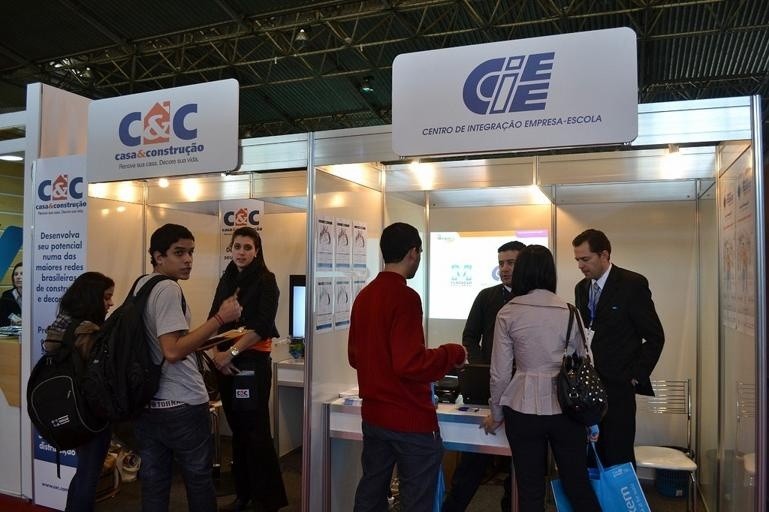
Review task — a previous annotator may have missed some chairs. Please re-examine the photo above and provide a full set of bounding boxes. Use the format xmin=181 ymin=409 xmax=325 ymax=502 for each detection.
xmin=634 ymin=377 xmax=712 ymax=512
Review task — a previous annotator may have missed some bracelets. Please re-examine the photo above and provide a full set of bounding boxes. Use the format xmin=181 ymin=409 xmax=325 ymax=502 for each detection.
xmin=212 ymin=312 xmax=226 ymax=329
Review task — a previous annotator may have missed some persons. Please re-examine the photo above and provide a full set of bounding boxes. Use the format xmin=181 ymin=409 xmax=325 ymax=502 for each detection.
xmin=439 ymin=240 xmax=528 ymax=512
xmin=347 ymin=221 xmax=468 ymax=511
xmin=477 ymin=244 xmax=601 ymax=512
xmin=572 ymin=229 xmax=665 ymax=474
xmin=206 ymin=226 xmax=290 ymax=511
xmin=35 ymin=270 xmax=120 ymax=511
xmin=0 ymin=262 xmax=24 ymax=328
xmin=127 ymin=222 xmax=243 ymax=512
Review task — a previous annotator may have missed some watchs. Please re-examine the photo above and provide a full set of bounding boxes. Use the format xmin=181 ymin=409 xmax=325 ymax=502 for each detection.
xmin=228 ymin=346 xmax=241 ymax=356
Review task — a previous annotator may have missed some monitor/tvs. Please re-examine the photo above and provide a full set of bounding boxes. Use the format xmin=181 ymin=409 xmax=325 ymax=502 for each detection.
xmin=459 ymin=364 xmax=492 ymax=406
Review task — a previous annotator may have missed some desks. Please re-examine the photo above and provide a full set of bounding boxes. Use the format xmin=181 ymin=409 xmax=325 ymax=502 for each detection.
xmin=274 ymin=357 xmax=305 ymax=463
xmin=324 ymin=396 xmax=518 ymax=512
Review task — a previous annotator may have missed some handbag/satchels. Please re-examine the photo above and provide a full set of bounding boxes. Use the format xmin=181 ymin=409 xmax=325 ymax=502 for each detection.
xmin=557 ymin=302 xmax=609 ymax=432
xmin=549 ymin=440 xmax=652 ymax=512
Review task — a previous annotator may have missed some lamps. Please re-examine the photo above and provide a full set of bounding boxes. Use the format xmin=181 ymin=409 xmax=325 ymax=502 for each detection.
xmin=295 ymin=27 xmax=308 ymax=45
xmin=80 ymin=59 xmax=95 ymax=80
xmin=362 ymin=75 xmax=374 ymax=94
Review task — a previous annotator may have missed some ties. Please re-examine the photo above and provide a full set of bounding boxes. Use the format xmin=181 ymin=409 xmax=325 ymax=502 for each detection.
xmin=589 ymin=282 xmax=600 ymax=329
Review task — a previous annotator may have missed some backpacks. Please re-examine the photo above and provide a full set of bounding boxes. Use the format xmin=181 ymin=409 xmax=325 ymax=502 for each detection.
xmin=80 ymin=274 xmax=187 ymax=426
xmin=25 ymin=319 xmax=112 ymax=450
